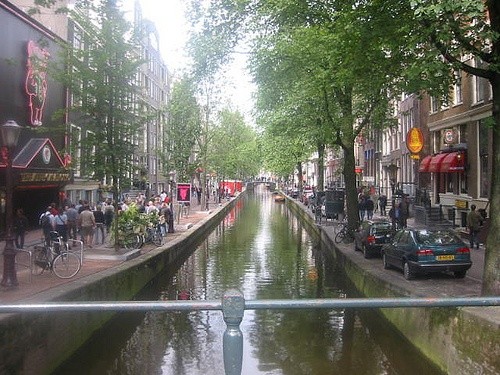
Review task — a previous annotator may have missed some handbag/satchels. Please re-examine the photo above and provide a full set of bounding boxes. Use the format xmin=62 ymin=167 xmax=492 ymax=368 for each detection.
xmin=473 ymin=224 xmax=480 ymax=231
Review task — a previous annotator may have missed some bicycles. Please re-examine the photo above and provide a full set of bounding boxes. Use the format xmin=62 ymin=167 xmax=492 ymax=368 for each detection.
xmin=124 ymin=219 xmax=165 ymax=250
xmin=334 ymin=222 xmax=359 ymax=244
xmin=30 ymin=231 xmax=82 ymax=279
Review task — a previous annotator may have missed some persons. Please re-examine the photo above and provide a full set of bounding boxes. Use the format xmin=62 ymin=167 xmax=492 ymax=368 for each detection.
xmin=359 ymin=192 xmax=373 ymax=221
xmin=118 ymin=192 xmax=171 ymax=236
xmin=13 ymin=208 xmax=29 ymax=248
xmin=388 ymin=197 xmax=409 ymax=229
xmin=468 ymin=204 xmax=483 ymax=249
xmin=378 ymin=195 xmax=387 ymax=216
xmin=39 ymin=199 xmax=115 ymax=256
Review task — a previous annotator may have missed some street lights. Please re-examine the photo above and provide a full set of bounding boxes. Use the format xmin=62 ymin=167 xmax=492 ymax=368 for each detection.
xmin=217 ymin=176 xmax=220 ymax=203
xmin=168 ymin=170 xmax=175 ymax=233
xmin=205 ymin=173 xmax=211 ymax=211
xmin=0 ymin=118 xmax=22 ymax=292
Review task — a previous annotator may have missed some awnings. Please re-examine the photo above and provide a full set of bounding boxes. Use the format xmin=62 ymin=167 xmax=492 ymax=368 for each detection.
xmin=418 ymin=151 xmax=465 ymax=172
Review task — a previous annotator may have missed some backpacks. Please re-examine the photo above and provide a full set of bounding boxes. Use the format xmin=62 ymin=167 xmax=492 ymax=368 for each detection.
xmin=41 ymin=213 xmax=51 ymax=228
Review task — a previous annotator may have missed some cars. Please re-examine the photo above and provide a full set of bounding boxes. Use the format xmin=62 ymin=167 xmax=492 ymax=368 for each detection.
xmin=281 ymin=183 xmax=345 ymax=219
xmin=380 ymin=224 xmax=473 ymax=280
xmin=353 ymin=219 xmax=400 ymax=259
